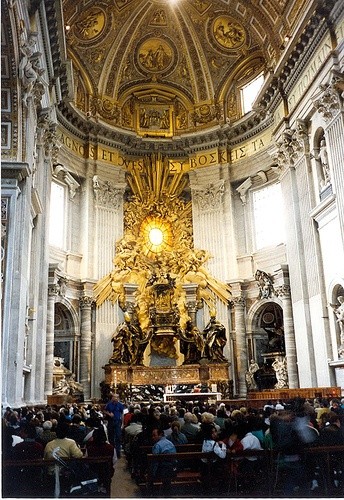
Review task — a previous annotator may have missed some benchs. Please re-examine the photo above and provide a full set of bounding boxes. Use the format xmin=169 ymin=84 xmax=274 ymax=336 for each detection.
xmin=2 ymin=435 xmax=344 ymax=499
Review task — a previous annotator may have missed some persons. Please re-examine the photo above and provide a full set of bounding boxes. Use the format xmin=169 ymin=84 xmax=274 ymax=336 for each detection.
xmin=13 ymin=28 xmax=344 ymax=209
xmin=105 ymin=309 xmax=231 ymax=367
xmin=1 ymin=403 xmax=112 ymax=498
xmin=122 ymin=396 xmax=344 ymax=498
xmin=103 ymin=393 xmax=126 ymax=460
xmin=51 ymin=373 xmax=84 ymax=396
xmin=246 ymin=268 xmax=344 ymax=391
